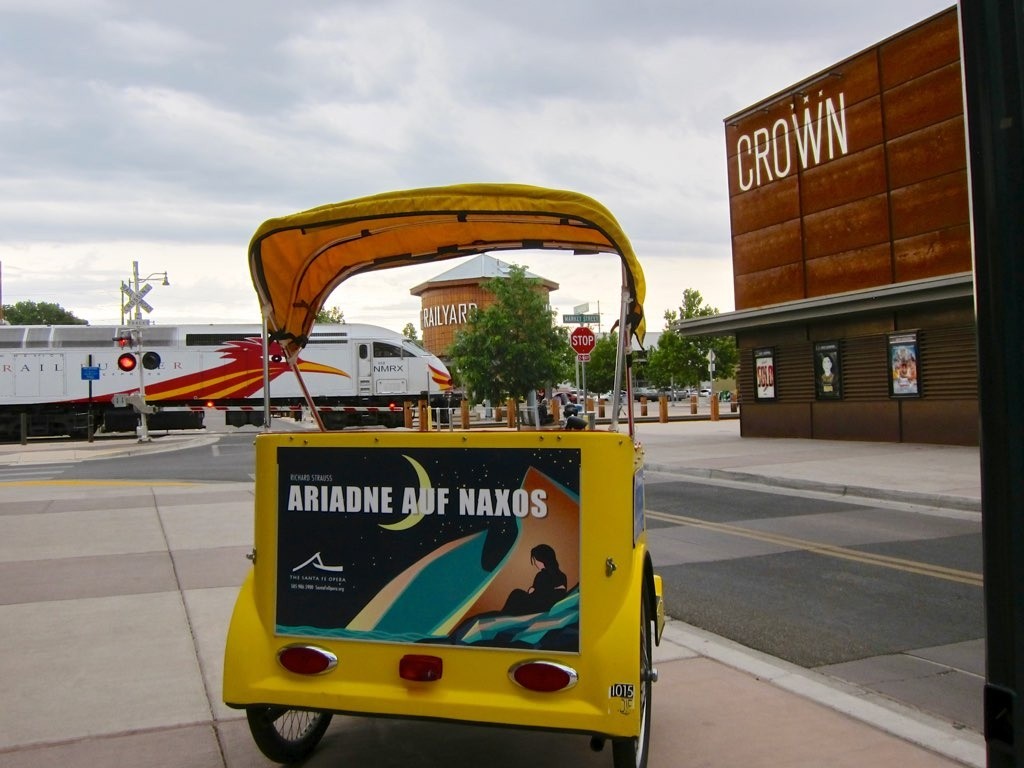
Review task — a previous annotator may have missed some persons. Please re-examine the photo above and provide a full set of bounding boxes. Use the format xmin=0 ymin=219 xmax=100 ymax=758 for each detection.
xmin=538 ymin=399 xmax=554 ymax=424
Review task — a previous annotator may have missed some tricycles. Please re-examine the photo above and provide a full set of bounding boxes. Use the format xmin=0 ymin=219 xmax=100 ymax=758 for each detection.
xmin=219 ymin=179 xmax=669 ymax=768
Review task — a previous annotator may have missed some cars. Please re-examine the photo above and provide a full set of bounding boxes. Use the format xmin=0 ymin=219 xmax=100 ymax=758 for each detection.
xmin=538 ymin=385 xmax=712 ymax=412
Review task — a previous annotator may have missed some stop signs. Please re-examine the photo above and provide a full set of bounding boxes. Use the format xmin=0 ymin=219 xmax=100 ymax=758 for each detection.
xmin=568 ymin=326 xmax=596 ymax=356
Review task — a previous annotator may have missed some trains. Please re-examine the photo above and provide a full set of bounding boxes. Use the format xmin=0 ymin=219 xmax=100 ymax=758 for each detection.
xmin=0 ymin=319 xmax=468 ymax=442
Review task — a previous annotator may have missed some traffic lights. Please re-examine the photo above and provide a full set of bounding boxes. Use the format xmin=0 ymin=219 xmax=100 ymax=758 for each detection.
xmin=141 ymin=350 xmax=161 ymax=371
xmin=116 ymin=353 xmax=137 ymax=372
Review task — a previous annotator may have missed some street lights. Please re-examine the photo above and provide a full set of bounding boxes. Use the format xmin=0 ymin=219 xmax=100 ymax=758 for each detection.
xmin=126 ymin=270 xmax=171 ymax=320
